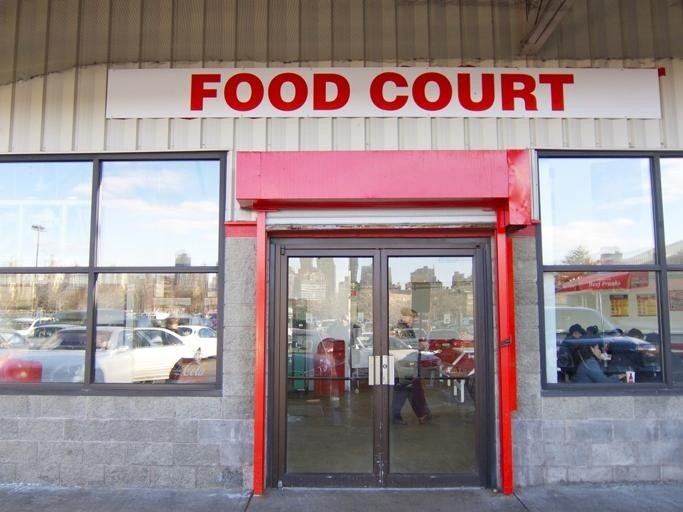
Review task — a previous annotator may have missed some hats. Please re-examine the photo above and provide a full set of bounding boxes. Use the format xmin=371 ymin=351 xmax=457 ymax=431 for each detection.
xmin=400 ymin=306 xmax=419 ymax=318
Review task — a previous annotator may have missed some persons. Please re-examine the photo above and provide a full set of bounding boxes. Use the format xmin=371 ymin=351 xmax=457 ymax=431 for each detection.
xmin=381 ymin=308 xmax=441 ymax=426
xmin=554 ymin=323 xmax=585 ymax=376
xmin=568 ymin=338 xmax=624 ymax=384
xmin=158 ymin=316 xmax=183 ymax=383
xmin=186 ymin=310 xmax=204 ymax=326
xmin=604 ymin=327 xmax=654 ymax=379
xmin=582 ymin=324 xmax=607 ymax=358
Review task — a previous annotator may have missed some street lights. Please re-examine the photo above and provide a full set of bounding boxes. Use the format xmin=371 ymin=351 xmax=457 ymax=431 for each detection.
xmin=32 ymin=226 xmax=43 ymax=284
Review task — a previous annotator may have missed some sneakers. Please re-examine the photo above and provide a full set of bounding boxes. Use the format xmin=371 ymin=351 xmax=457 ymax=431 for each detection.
xmin=418 ymin=413 xmax=439 ymax=424
xmin=392 ymin=415 xmax=408 ymax=426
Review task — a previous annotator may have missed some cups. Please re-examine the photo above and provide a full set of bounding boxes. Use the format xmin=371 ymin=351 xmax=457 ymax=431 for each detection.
xmin=626 ymin=371 xmax=635 ymax=384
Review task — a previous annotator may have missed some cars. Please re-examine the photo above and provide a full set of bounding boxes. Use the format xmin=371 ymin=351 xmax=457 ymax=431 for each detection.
xmin=1 ymin=308 xmax=217 ymax=383
xmin=556 ymin=307 xmax=661 ymax=380
xmin=287 ymin=309 xmax=476 ymax=393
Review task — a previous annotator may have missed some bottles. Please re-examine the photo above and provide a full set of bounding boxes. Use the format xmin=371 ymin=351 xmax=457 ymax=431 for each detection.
xmin=629 ymin=373 xmax=633 ymax=384
